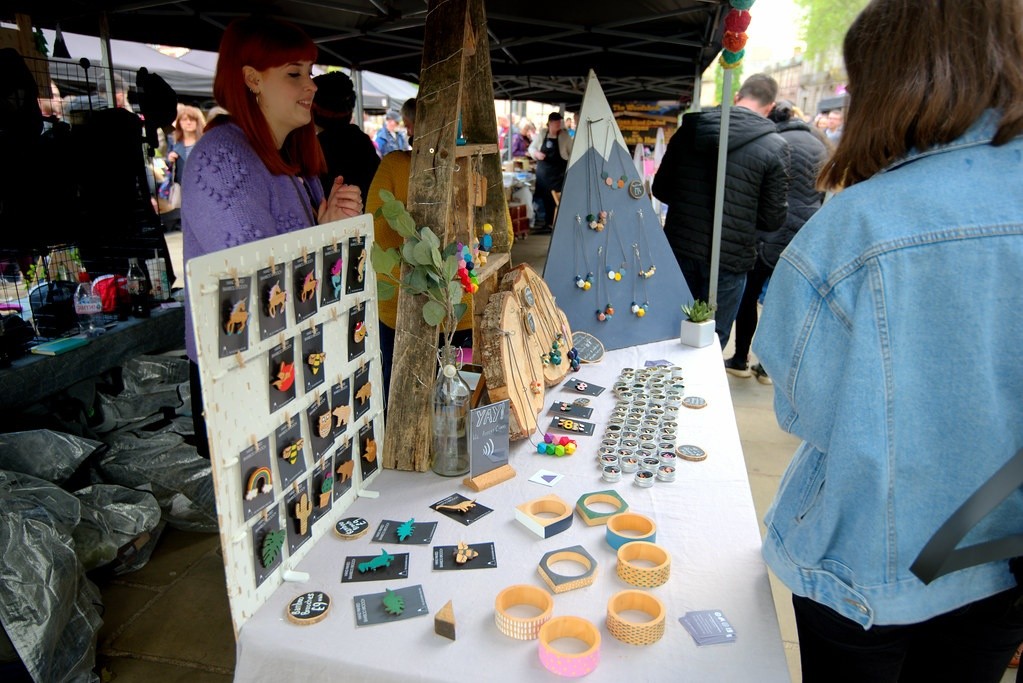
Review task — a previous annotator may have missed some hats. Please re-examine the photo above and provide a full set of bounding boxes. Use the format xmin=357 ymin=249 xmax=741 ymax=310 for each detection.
xmin=386 ymin=112 xmax=401 ymax=122
xmin=310 ymin=71 xmax=356 ymax=129
xmin=549 ymin=112 xmax=564 ymax=120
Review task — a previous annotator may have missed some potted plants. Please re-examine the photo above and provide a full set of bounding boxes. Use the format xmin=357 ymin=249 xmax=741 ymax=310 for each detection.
xmin=681 ymin=300 xmax=716 ymax=348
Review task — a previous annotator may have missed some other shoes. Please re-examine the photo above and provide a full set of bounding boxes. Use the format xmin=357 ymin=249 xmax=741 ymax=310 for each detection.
xmin=724 ymin=357 xmax=751 ymax=378
xmin=750 ymin=363 xmax=772 ymax=385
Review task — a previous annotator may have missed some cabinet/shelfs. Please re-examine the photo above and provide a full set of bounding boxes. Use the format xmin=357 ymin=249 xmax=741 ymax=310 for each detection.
xmin=381 ymin=0 xmax=514 ymax=474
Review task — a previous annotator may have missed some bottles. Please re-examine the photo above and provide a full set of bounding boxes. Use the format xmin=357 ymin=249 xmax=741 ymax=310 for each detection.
xmin=126 ymin=258 xmax=151 ymax=319
xmin=73 ymin=272 xmax=106 ymax=337
xmin=144 ymin=258 xmax=170 ymax=300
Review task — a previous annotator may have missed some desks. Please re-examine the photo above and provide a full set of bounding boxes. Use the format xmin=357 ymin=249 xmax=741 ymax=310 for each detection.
xmin=235 ymin=331 xmax=788 ymax=683
xmin=0 ymin=288 xmax=186 ymax=412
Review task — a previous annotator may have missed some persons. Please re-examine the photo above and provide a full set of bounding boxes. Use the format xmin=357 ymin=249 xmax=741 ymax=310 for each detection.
xmin=364 ymin=149 xmax=475 ymax=433
xmin=348 ymin=105 xmax=408 ymax=157
xmin=494 ymin=109 xmax=579 ymax=237
xmin=649 ymin=73 xmax=851 ymax=385
xmin=402 ymin=98 xmax=419 ymax=149
xmin=756 ymin=1 xmax=1023 ymax=683
xmin=310 ymin=72 xmax=381 ymax=206
xmin=180 ymin=19 xmax=361 ymax=467
xmin=37 ymin=65 xmax=234 ymax=235
